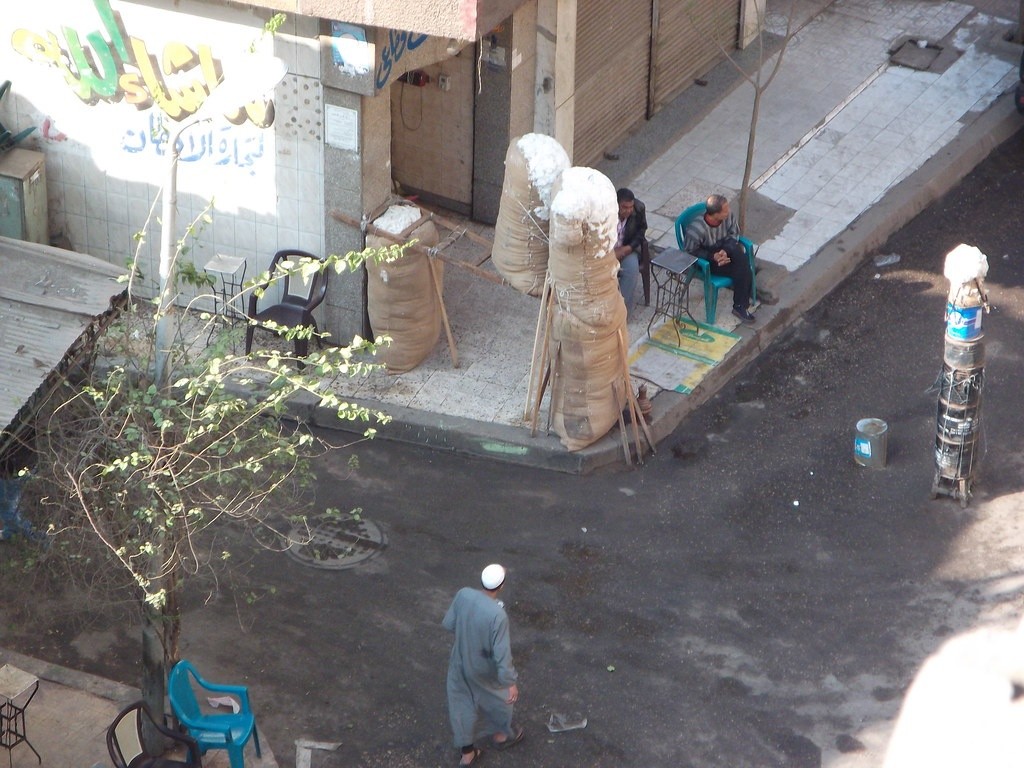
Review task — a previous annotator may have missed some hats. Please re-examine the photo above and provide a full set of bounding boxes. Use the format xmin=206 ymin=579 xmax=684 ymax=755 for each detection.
xmin=480 ymin=563 xmax=506 ymax=590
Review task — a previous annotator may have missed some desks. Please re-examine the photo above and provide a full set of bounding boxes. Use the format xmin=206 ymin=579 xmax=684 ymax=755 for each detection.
xmin=0 ymin=663 xmax=41 ymax=768
xmin=647 ymin=247 xmax=699 ymax=348
xmin=204 ymin=254 xmax=247 ymax=355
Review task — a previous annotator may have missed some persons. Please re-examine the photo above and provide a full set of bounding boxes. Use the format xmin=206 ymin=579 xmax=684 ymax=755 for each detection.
xmin=684 ymin=195 xmax=779 ymax=323
xmin=613 ymin=188 xmax=648 ymax=277
xmin=443 ymin=564 xmax=523 ymax=768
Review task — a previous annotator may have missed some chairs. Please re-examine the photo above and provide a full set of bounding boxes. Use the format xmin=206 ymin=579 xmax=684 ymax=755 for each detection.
xmin=168 ymin=660 xmax=261 ymax=768
xmin=675 ymin=202 xmax=756 ymax=324
xmin=106 ymin=701 xmax=203 ymax=768
xmin=246 ymin=250 xmax=329 ymax=376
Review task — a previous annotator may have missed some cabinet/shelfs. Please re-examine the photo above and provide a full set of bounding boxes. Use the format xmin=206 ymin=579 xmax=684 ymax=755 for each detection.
xmin=0 ymin=148 xmax=49 ymax=245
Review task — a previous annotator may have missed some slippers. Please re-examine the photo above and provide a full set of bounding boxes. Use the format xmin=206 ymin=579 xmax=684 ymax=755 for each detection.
xmin=459 ymin=748 xmax=483 ymax=767
xmin=493 ymin=723 xmax=526 ymax=750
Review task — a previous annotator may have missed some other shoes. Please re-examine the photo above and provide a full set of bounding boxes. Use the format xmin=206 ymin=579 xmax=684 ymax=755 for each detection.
xmin=732 ymin=303 xmax=756 ymax=322
